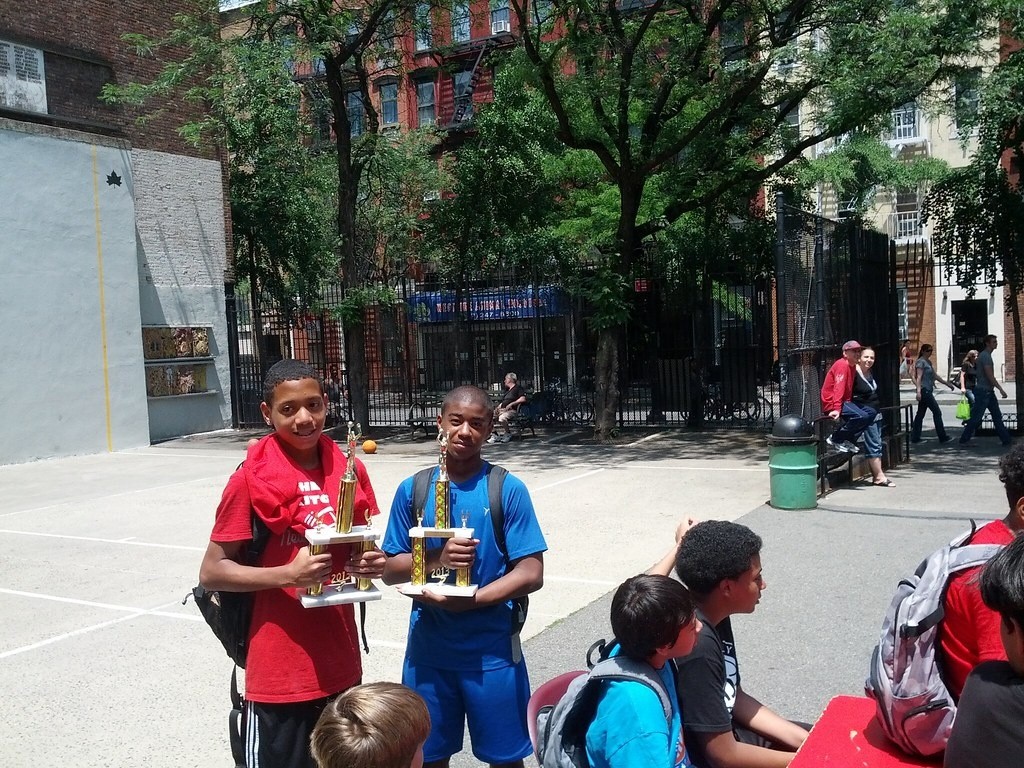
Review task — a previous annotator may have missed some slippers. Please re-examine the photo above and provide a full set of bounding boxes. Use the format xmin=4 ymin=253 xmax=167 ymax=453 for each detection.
xmin=872 ymin=478 xmax=896 ymax=487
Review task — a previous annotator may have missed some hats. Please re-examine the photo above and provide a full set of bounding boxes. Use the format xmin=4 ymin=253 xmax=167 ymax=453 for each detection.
xmin=841 ymin=340 xmax=864 ymax=351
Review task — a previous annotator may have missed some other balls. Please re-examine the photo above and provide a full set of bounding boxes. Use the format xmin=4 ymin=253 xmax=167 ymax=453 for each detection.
xmin=362 ymin=440 xmax=377 ymax=453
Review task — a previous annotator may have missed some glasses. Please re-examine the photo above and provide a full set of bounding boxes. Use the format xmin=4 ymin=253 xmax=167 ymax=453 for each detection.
xmin=926 ymin=349 xmax=933 ymax=352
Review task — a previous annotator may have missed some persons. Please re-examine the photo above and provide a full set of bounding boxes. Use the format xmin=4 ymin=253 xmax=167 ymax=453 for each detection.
xmin=322 ymin=364 xmax=348 ymax=421
xmin=200 ymin=358 xmax=385 ymax=768
xmin=437 ymin=429 xmax=449 ymax=478
xmin=381 ymin=387 xmax=548 ymax=768
xmin=911 ymin=344 xmax=954 ymax=443
xmin=958 ymin=335 xmax=1017 ymax=446
xmin=821 ymin=340 xmax=896 ymax=487
xmin=899 ymin=339 xmax=917 ymax=386
xmin=309 ymin=682 xmax=431 ymax=768
xmin=585 ymin=512 xmax=810 ymax=768
xmin=933 ymin=440 xmax=1024 ymax=708
xmin=486 ymin=373 xmax=526 ymax=444
xmin=959 ymin=350 xmax=979 ymax=427
xmin=346 ymin=421 xmax=362 ymax=473
xmin=944 ymin=530 xmax=1024 ymax=768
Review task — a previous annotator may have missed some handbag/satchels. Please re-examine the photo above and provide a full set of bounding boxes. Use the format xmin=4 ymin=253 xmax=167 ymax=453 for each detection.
xmin=899 ymin=357 xmax=908 ymax=376
xmin=951 ymin=361 xmax=966 ymax=390
xmin=956 ymin=391 xmax=971 ymax=420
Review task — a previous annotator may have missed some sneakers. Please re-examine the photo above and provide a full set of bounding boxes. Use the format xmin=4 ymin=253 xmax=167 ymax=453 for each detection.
xmin=501 ymin=431 xmax=512 ymax=443
xmin=826 ymin=434 xmax=860 ymax=454
xmin=487 ymin=433 xmax=500 ymax=443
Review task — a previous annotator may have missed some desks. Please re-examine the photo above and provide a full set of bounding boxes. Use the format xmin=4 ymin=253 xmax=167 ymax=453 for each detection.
xmin=789 ymin=693 xmax=943 ymax=768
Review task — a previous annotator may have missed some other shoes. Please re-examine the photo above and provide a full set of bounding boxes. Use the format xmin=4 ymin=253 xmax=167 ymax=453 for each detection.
xmin=911 ymin=440 xmax=927 ymax=444
xmin=939 ymin=436 xmax=955 ymax=443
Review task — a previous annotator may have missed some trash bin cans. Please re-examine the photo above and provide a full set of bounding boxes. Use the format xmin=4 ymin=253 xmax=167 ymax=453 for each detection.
xmin=765 ymin=413 xmax=819 ymax=511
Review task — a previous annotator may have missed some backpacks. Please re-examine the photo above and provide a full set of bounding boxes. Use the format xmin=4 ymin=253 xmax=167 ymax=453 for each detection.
xmin=864 ymin=519 xmax=1011 ymax=755
xmin=536 ymin=639 xmax=678 ymax=768
xmin=180 ymin=460 xmax=369 ymax=669
xmin=411 ymin=464 xmax=530 ymax=634
